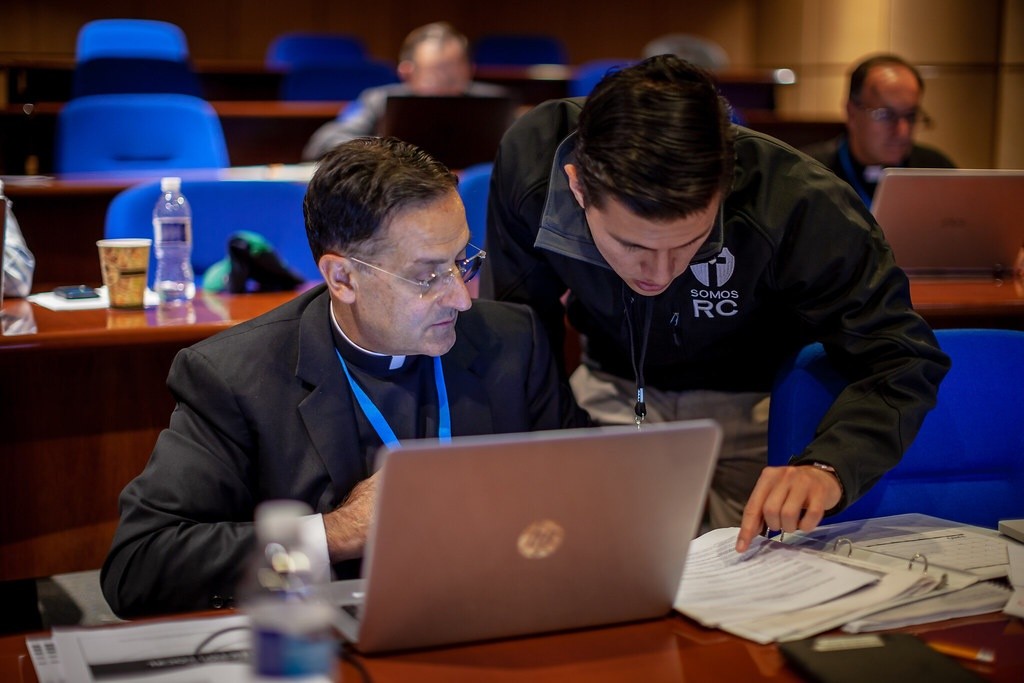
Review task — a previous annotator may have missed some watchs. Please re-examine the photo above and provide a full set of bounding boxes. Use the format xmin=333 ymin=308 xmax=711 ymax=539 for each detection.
xmin=811 ymin=461 xmax=844 ymax=498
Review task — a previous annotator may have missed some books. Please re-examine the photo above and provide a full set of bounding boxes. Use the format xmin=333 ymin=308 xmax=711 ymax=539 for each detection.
xmin=55 ymin=285 xmax=100 ymax=300
xmin=671 ymin=512 xmax=1024 ymax=683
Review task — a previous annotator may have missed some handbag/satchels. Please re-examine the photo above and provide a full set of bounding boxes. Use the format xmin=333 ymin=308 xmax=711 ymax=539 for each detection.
xmin=201 ymin=231 xmax=305 ymax=293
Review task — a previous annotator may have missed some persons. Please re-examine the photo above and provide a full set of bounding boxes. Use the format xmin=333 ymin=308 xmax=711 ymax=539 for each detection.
xmin=97 ymin=135 xmax=602 ymax=619
xmin=482 ymin=51 xmax=952 ymax=553
xmin=302 ymin=21 xmax=508 ymax=161
xmin=813 ymin=54 xmax=959 ymax=210
xmin=0 ymin=180 xmax=36 ymax=298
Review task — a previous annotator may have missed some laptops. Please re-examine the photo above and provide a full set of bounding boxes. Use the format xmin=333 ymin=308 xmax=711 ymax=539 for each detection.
xmin=307 ymin=418 xmax=723 ymax=655
xmin=864 ymin=168 xmax=1024 ymax=279
xmin=380 ymin=93 xmax=512 ymax=177
xmin=710 ymin=80 xmax=777 ymax=131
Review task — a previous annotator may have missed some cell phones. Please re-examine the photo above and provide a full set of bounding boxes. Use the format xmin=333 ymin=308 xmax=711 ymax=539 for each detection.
xmin=55 ymin=282 xmax=97 ymax=300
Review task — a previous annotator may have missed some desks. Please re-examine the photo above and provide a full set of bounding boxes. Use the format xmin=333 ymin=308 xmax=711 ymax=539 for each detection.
xmin=0 ymin=180 xmax=140 ymax=286
xmin=1 ymin=274 xmax=1024 ymax=545
xmin=0 ymin=101 xmax=543 ymax=181
xmin=1 ymin=601 xmax=1024 ymax=683
xmin=0 ymin=52 xmax=587 ymax=102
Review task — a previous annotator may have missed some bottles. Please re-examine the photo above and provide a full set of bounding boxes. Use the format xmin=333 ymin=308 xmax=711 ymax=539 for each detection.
xmin=152 ymin=177 xmax=195 ymax=310
xmin=248 ymin=498 xmax=337 ymax=683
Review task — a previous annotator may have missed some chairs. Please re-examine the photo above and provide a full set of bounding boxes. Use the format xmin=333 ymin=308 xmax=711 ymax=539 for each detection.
xmin=767 ymin=329 xmax=1024 ymax=538
xmin=58 ymin=21 xmax=737 ymax=292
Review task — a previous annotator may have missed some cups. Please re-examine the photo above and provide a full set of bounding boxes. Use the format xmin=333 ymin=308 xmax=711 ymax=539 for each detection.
xmin=96 ymin=238 xmax=152 ymax=310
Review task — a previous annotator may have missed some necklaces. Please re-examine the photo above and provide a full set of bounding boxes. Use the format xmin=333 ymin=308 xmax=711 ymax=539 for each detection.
xmin=621 ymin=275 xmax=655 ymax=424
xmin=840 ymin=136 xmax=872 ymax=210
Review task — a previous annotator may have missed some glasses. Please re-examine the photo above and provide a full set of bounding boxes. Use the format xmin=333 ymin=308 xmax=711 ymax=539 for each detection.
xmin=852 ymin=101 xmax=920 ymax=124
xmin=324 ymin=243 xmax=486 ymax=301
